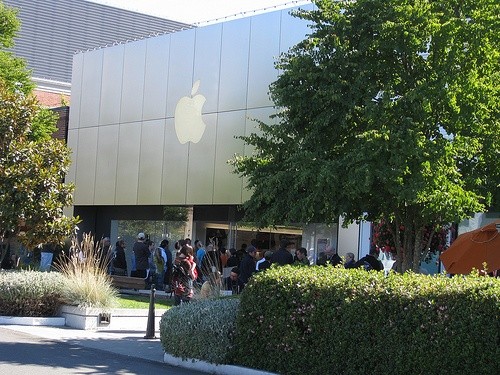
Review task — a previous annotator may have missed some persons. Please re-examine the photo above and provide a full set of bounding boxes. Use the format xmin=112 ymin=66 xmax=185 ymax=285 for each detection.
xmin=70 ymin=232 xmax=356 ymax=306
xmin=356 ymin=245 xmax=383 ymax=271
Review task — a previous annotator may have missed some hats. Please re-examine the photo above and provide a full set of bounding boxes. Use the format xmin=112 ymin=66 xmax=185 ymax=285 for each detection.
xmin=138 ymin=232 xmax=145 ymax=238
xmin=248 ymin=246 xmax=256 ymax=251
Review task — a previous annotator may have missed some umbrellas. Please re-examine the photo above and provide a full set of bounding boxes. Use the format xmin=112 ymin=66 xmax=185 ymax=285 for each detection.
xmin=440 ymin=224 xmax=500 ymax=277
